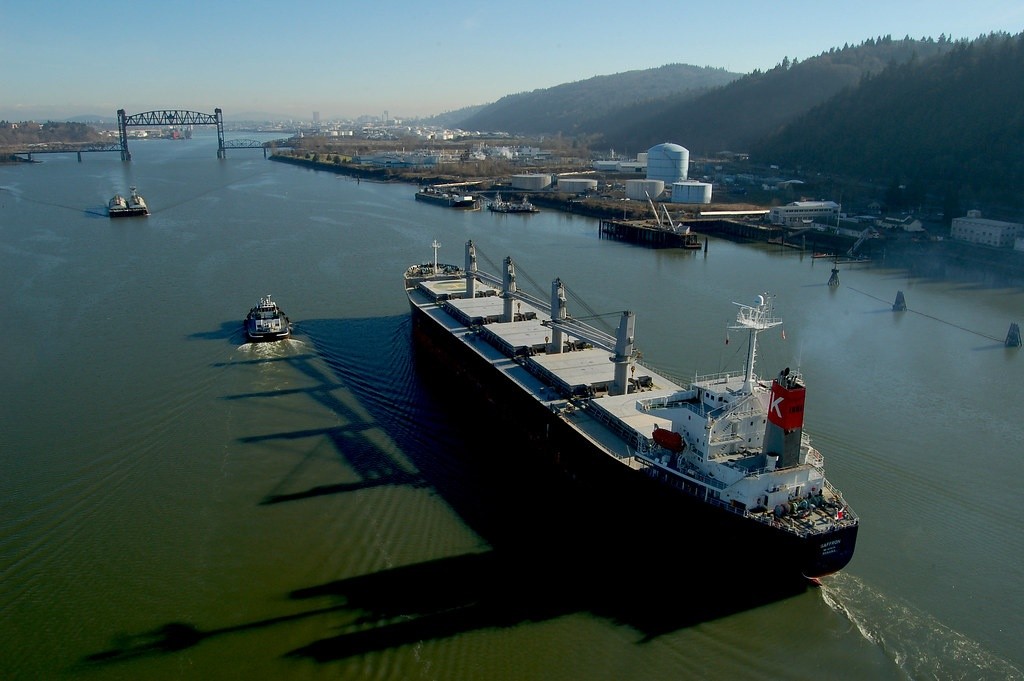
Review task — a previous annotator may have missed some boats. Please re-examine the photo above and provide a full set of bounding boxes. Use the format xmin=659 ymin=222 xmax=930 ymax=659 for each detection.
xmin=487 ymin=191 xmax=540 ymax=214
xmin=244 ymin=295 xmax=292 ymax=342
xmin=108 ymin=187 xmax=149 ymax=216
xmin=415 ymin=185 xmax=476 ymax=207
xmin=403 ymin=240 xmax=860 ymax=578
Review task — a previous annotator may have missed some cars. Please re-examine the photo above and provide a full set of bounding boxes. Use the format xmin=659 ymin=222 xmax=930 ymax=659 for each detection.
xmin=37 ymin=143 xmax=47 ymax=147
xmin=868 ymin=231 xmax=880 ymax=239
xmin=28 ymin=144 xmax=35 ymax=148
